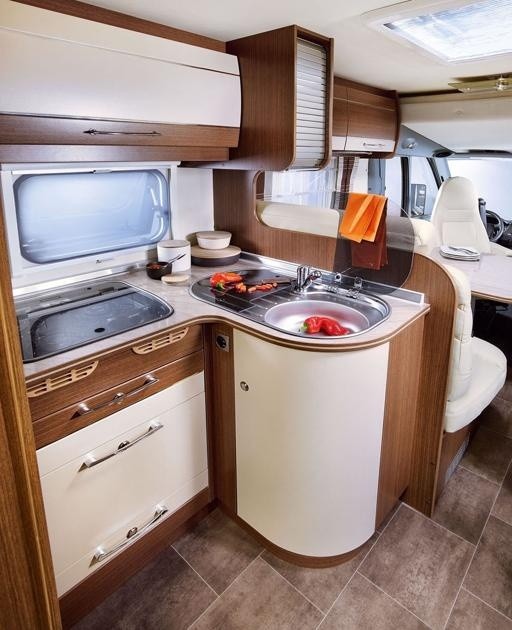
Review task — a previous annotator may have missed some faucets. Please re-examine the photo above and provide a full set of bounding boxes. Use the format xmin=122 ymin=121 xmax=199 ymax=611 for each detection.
xmin=298 ymin=266 xmax=321 ymax=290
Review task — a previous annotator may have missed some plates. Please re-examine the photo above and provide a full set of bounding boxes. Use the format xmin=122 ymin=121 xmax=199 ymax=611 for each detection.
xmin=439 ymin=244 xmax=480 ymax=263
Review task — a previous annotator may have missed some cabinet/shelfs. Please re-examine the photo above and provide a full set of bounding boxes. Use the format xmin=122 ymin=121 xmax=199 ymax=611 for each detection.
xmin=332 ymin=86 xmax=398 ymax=153
xmin=1 ymin=2 xmax=245 ymax=149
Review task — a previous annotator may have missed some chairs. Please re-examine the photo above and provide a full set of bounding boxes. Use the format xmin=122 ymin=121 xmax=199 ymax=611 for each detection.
xmin=384 ymin=179 xmax=512 ymax=436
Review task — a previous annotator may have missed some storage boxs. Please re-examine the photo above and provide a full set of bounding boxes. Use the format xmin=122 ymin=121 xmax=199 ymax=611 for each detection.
xmin=230 ymin=329 xmax=394 ymax=559
xmin=34 ymin=350 xmax=213 ymax=605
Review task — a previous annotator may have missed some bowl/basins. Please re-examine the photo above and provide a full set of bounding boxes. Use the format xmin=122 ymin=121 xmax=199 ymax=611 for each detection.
xmin=146 ymin=262 xmax=171 ymax=280
xmin=197 ymin=236 xmax=232 ymax=251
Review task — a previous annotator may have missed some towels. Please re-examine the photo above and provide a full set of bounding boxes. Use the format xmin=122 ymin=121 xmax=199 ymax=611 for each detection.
xmin=337 ymin=193 xmax=391 ymax=272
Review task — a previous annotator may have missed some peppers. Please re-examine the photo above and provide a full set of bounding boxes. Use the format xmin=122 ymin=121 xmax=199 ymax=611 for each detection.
xmin=209 ymin=271 xmax=242 ymax=290
xmin=299 ymin=316 xmax=348 ymax=335
